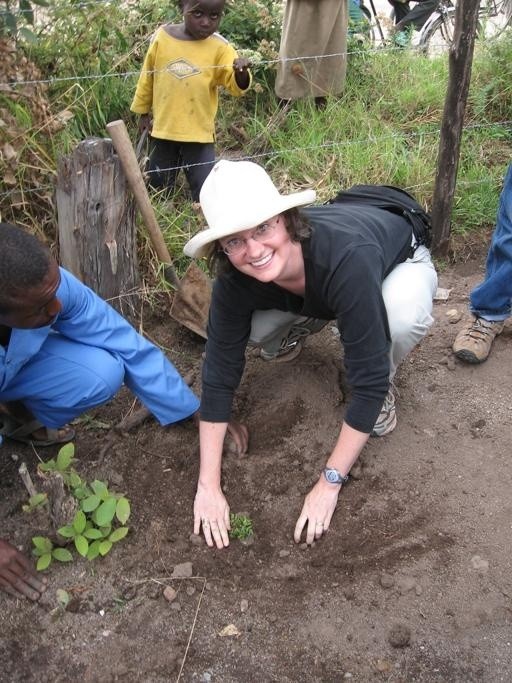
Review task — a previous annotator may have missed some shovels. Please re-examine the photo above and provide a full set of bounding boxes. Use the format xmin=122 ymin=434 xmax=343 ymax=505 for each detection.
xmin=106 ymin=117 xmax=215 ymax=340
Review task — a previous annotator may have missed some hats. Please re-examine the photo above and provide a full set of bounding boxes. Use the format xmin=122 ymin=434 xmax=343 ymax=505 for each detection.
xmin=183 ymin=156 xmax=317 ymax=260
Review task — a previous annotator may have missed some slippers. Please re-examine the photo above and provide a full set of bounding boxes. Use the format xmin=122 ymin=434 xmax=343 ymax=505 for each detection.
xmin=1 ymin=419 xmax=77 ymax=447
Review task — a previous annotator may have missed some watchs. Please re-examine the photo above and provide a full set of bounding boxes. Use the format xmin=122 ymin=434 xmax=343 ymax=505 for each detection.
xmin=322 ymin=466 xmax=347 ymax=484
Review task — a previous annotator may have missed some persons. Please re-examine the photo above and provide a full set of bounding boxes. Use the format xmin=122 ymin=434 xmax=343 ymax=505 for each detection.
xmin=131 ymin=2 xmax=253 ymax=201
xmin=388 ymin=0 xmax=440 ymax=47
xmin=275 ymin=0 xmax=348 ymax=113
xmin=0 ymin=219 xmax=250 ymax=601
xmin=452 ymin=159 xmax=512 ymax=364
xmin=183 ymin=158 xmax=438 ymax=551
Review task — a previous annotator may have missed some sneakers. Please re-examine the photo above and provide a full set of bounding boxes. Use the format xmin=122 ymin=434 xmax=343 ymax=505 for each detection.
xmin=370 ymin=383 xmax=399 ymax=439
xmin=452 ymin=312 xmax=506 ymax=365
xmin=258 ymin=319 xmax=329 ymax=365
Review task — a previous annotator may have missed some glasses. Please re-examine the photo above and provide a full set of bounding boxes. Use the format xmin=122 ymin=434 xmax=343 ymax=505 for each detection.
xmin=217 ymin=216 xmax=283 ymax=256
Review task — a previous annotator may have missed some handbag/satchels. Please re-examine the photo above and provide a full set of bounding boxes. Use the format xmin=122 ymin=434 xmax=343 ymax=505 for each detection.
xmin=332 ymin=182 xmax=437 ymax=253
